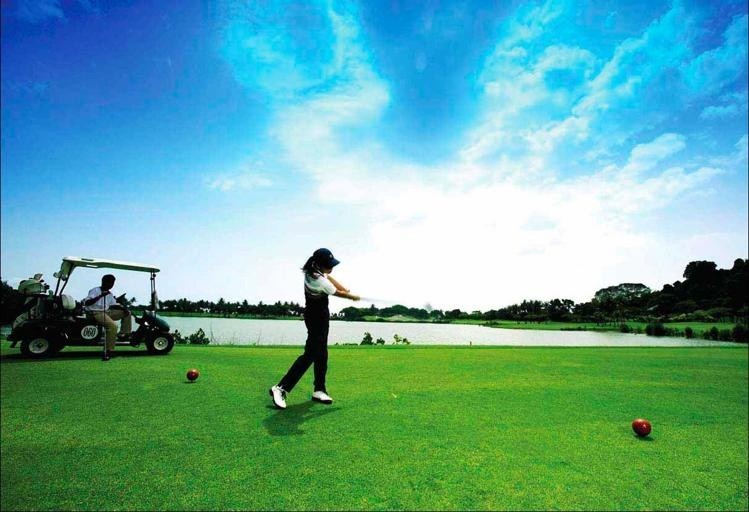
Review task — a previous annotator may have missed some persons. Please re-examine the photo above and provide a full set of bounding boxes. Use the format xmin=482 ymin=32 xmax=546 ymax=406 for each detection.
xmin=84 ymin=273 xmax=132 ymax=360
xmin=269 ymin=248 xmax=360 ymax=410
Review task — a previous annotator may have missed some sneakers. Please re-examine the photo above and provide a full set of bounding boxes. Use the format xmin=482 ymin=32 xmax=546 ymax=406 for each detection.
xmin=269 ymin=385 xmax=288 ymax=409
xmin=103 ymin=331 xmax=131 ymax=363
xmin=311 ymin=389 xmax=333 ymax=404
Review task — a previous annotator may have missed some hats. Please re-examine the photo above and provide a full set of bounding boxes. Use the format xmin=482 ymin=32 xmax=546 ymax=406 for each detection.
xmin=313 ymin=247 xmax=340 ymax=270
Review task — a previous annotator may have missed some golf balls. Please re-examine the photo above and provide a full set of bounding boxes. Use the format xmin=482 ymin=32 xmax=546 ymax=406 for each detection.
xmin=187 ymin=369 xmax=198 ymax=379
xmin=632 ymin=418 xmax=651 ymax=437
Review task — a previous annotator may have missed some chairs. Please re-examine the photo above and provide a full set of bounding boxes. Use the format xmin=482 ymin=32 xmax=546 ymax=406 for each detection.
xmin=61 ymin=293 xmax=107 ymax=335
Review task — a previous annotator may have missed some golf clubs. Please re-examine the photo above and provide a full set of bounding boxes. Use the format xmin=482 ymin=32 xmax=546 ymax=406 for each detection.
xmin=102 ymin=297 xmax=109 ymax=360
